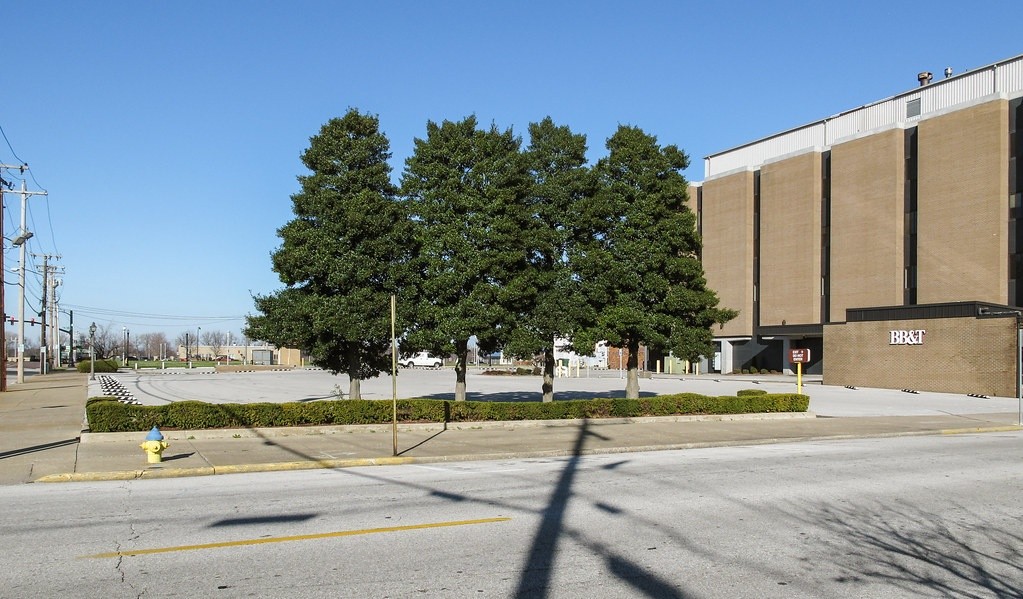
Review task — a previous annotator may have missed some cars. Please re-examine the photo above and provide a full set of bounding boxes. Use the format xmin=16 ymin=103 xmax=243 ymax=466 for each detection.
xmin=396 ymin=350 xmax=443 ymax=369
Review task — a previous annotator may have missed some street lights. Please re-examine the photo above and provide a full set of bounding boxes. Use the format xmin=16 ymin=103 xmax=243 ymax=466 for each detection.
xmin=123 ymin=326 xmax=127 ymax=367
xmin=196 ymin=326 xmax=201 ymax=361
xmin=88 ymin=322 xmax=97 ymax=380
xmin=10 ymin=267 xmax=46 ymax=373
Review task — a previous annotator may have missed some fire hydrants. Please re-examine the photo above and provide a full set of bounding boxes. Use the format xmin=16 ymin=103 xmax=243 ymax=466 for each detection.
xmin=138 ymin=424 xmax=171 ymax=463
